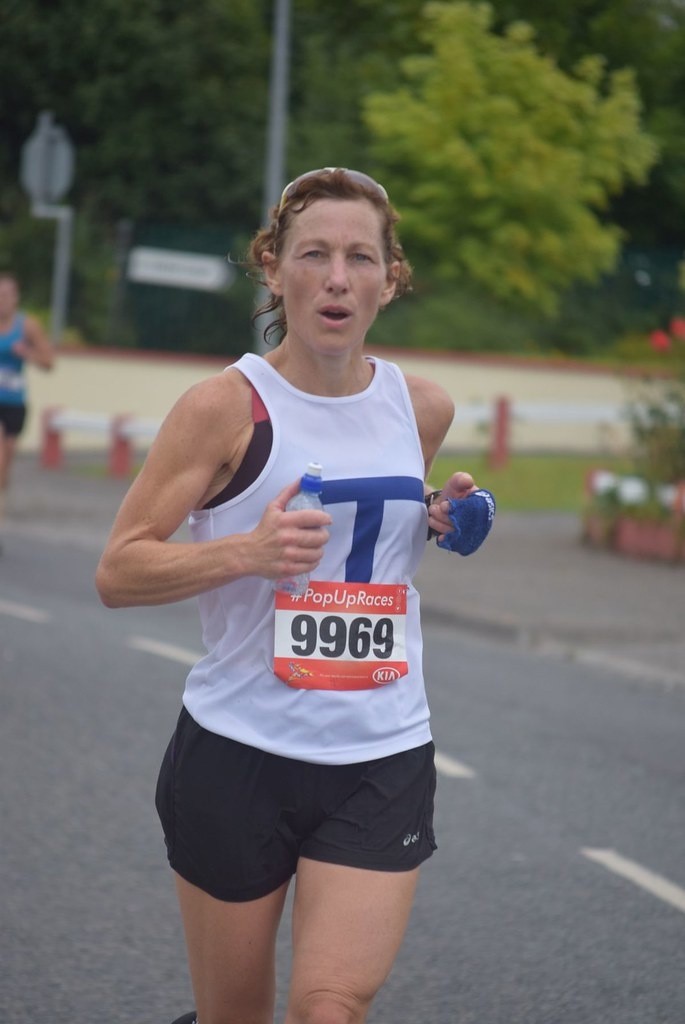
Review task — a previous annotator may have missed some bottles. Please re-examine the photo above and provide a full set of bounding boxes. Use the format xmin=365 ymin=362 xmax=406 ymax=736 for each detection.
xmin=268 ymin=461 xmax=324 ymax=595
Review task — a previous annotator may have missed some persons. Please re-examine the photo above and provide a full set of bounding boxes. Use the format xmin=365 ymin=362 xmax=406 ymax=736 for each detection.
xmin=0 ymin=269 xmax=56 ymax=490
xmin=94 ymin=168 xmax=496 ymax=1024
